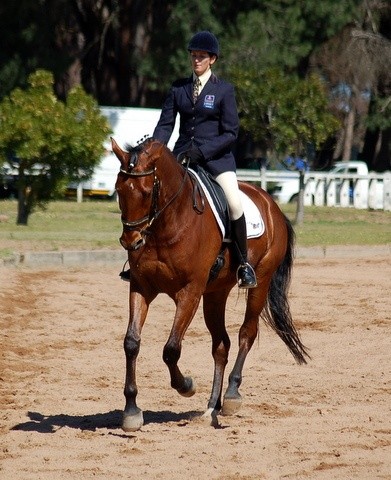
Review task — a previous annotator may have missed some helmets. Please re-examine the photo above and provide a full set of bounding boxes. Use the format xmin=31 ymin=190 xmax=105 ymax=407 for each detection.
xmin=188 ymin=31 xmax=219 ymax=54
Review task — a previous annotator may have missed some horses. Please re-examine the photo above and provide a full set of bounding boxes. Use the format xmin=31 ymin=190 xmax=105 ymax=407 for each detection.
xmin=110 ymin=137 xmax=312 ymax=432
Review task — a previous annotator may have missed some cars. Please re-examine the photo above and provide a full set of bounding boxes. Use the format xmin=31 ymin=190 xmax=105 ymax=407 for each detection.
xmin=277 ymin=159 xmax=368 ymax=206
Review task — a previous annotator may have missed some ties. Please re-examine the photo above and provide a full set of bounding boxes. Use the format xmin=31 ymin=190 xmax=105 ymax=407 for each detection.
xmin=193 ymin=78 xmax=201 ymax=104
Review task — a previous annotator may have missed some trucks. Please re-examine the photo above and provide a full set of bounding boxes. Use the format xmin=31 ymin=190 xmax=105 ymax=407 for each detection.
xmin=0 ymin=104 xmax=185 ymax=194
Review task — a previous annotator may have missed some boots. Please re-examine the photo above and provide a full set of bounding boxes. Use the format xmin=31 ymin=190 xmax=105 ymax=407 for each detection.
xmin=230 ymin=212 xmax=256 ymax=285
xmin=120 ymin=269 xmax=130 ymax=279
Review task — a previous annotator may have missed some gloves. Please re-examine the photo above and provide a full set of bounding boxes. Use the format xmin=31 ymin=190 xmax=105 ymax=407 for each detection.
xmin=186 ymin=149 xmax=203 ymax=159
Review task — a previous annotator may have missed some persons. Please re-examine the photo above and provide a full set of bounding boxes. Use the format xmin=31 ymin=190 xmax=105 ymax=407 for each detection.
xmin=152 ymin=32 xmax=258 ymax=288
